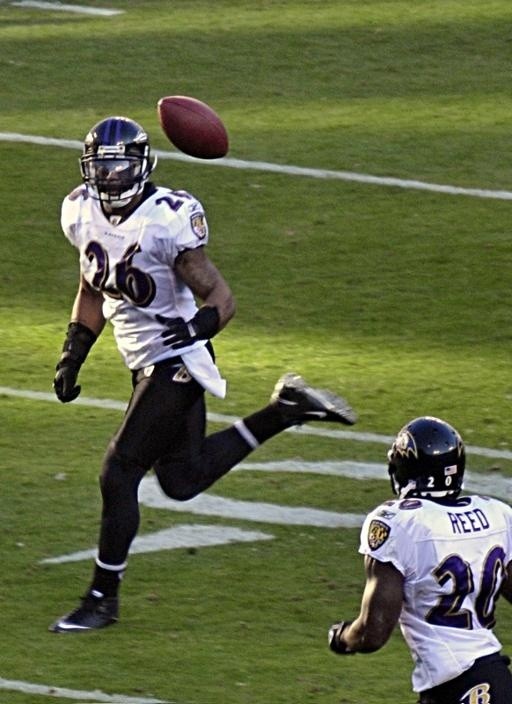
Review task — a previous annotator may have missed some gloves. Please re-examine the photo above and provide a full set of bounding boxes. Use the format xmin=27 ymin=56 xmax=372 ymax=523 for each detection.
xmin=54 ymin=351 xmax=84 ymax=403
xmin=154 ymin=305 xmax=222 ymax=350
xmin=327 ymin=621 xmax=354 ymax=656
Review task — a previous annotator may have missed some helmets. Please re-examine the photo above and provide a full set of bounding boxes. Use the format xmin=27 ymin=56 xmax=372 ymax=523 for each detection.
xmin=388 ymin=415 xmax=467 ymax=498
xmin=79 ymin=116 xmax=148 ymax=184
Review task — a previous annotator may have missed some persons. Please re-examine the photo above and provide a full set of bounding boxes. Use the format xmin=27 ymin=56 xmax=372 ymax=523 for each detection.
xmin=48 ymin=117 xmax=358 ymax=634
xmin=327 ymin=415 xmax=512 ymax=704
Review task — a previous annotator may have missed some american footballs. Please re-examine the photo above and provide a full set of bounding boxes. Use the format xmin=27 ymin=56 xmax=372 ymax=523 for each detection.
xmin=158 ymin=95 xmax=228 ymax=159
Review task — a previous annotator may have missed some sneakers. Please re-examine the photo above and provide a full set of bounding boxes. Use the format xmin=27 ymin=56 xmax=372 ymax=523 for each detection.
xmin=270 ymin=373 xmax=360 ymax=425
xmin=49 ymin=587 xmax=119 ymax=633
